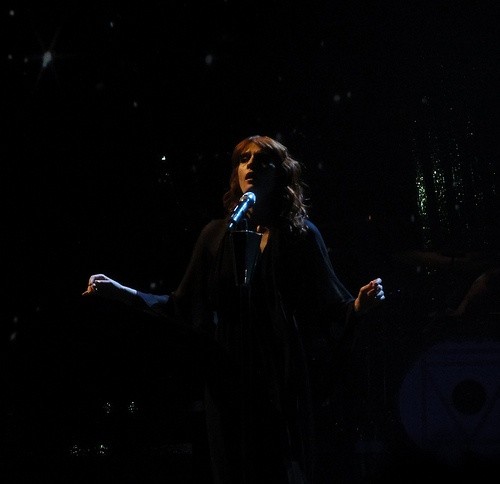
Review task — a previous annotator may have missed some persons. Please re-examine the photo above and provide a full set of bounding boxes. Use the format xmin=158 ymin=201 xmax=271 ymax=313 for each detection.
xmin=82 ymin=133 xmax=397 ymax=484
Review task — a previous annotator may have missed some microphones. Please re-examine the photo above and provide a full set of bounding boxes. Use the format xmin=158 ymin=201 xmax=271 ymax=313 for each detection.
xmin=227 ymin=192 xmax=256 ymax=231
